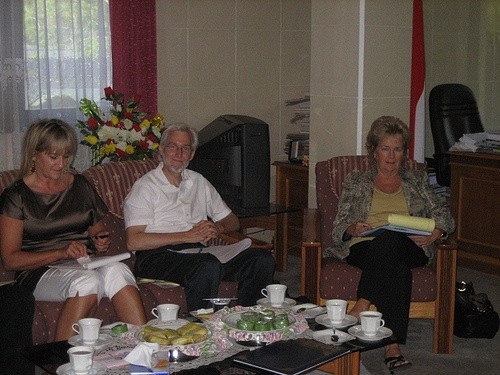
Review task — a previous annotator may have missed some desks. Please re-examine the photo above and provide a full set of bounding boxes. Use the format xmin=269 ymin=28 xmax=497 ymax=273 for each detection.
xmin=16 ymin=296 xmax=397 ymax=375
xmin=220 ymin=217 xmax=300 ymax=249
xmin=272 ymin=161 xmax=309 ymax=274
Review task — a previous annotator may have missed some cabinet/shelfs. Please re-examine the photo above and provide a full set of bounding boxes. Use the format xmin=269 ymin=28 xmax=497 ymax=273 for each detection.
xmin=445 ymin=151 xmax=500 ymax=275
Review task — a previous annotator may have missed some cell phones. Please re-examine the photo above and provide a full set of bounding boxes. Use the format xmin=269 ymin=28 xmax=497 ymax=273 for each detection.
xmin=91 ymin=234 xmax=109 ymax=249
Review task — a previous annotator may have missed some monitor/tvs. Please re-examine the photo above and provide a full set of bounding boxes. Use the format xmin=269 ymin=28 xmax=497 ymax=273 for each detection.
xmin=184 ymin=114 xmax=272 ymax=220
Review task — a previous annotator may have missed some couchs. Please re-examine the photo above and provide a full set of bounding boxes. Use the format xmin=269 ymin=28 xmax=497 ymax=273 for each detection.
xmin=0 ymin=158 xmax=274 ymax=347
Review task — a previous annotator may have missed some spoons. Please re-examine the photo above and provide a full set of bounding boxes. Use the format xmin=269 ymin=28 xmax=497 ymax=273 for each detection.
xmin=331 ymin=325 xmax=339 ymax=343
xmin=295 ymin=303 xmax=327 ymax=314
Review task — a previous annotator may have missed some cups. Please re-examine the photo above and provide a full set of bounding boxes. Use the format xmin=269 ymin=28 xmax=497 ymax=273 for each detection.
xmin=359 ymin=311 xmax=385 ymax=336
xmin=152 ymin=304 xmax=180 ymax=321
xmin=209 ymin=297 xmax=230 ymax=313
xmin=326 ymin=299 xmax=349 ymax=323
xmin=71 ymin=318 xmax=102 ymax=343
xmin=262 ymin=284 xmax=287 ymax=307
xmin=67 ymin=346 xmax=94 ymax=374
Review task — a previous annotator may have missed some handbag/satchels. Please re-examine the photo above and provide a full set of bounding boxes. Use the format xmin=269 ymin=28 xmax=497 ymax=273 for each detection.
xmin=454 ymin=281 xmax=500 ymax=339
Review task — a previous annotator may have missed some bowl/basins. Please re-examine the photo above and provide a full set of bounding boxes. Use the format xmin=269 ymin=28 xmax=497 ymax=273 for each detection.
xmin=222 ymin=312 xmax=297 ymax=346
xmin=133 ymin=330 xmax=200 ymax=362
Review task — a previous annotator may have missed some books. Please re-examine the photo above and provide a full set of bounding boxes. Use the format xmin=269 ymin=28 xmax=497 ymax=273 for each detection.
xmin=48 ymin=252 xmax=131 ymax=270
xmin=360 ymin=224 xmax=433 ymax=237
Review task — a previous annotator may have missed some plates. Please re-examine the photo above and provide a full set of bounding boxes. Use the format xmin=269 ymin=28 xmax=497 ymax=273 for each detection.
xmin=57 ymin=363 xmax=98 ymax=375
xmin=147 ymin=317 xmax=190 ymax=328
xmin=311 ymin=329 xmax=356 ymax=344
xmin=289 ymin=303 xmax=326 ymax=319
xmin=102 ymin=322 xmax=135 ymax=337
xmin=313 ymin=313 xmax=358 ymax=329
xmin=256 ymin=298 xmax=297 ymax=311
xmin=67 ymin=334 xmax=113 ymax=349
xmin=347 ymin=324 xmax=393 ymax=341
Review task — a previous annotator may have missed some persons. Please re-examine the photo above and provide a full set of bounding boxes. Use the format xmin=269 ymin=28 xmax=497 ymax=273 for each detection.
xmin=123 ymin=123 xmax=276 ymax=317
xmin=322 ymin=116 xmax=456 ymax=375
xmin=0 ymin=119 xmax=148 ymax=341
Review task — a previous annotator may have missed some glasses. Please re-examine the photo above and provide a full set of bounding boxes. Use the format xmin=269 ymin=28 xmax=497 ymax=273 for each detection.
xmin=164 ymin=143 xmax=194 ymax=153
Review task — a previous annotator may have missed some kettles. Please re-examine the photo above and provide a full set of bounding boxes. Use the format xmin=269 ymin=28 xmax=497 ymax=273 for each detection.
xmin=288 ymin=139 xmax=304 ymax=164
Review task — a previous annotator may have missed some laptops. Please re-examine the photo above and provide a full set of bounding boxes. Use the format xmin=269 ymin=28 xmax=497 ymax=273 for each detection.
xmin=226 ymin=338 xmax=351 ymax=375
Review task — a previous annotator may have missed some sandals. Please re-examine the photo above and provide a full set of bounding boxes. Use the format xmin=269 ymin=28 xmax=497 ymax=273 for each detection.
xmin=384 ymin=355 xmax=412 ymax=370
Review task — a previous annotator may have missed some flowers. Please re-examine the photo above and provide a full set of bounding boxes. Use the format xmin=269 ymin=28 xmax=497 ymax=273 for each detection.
xmin=73 ymin=87 xmax=161 ymax=167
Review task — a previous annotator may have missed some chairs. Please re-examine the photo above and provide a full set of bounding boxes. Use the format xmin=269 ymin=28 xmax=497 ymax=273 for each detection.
xmin=429 ymin=84 xmax=484 ymax=188
xmin=300 ymin=155 xmax=458 ymax=354
